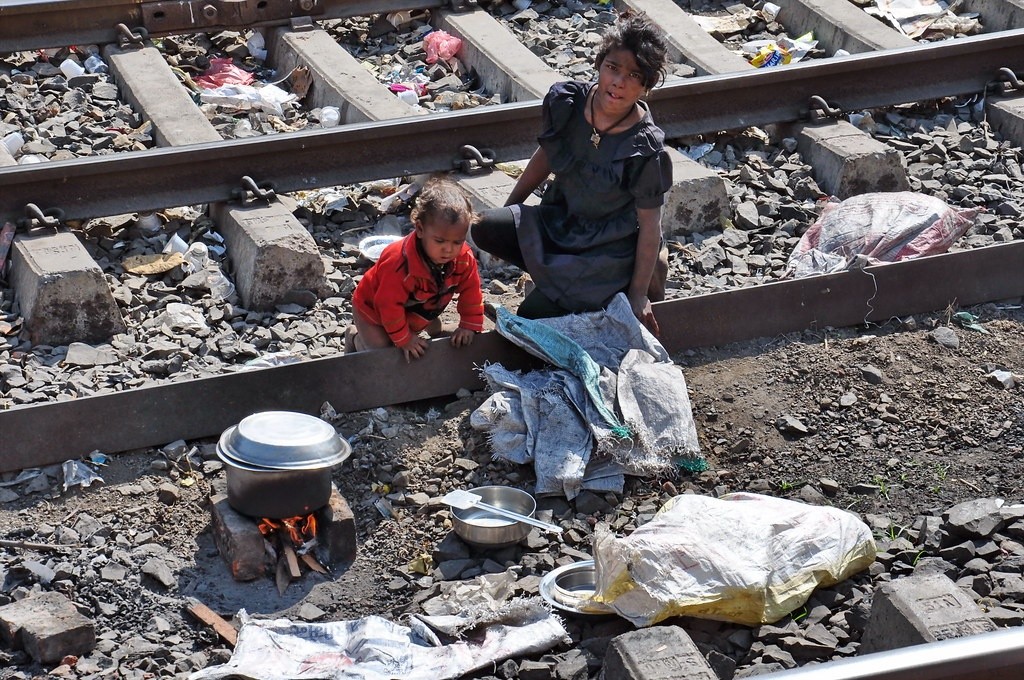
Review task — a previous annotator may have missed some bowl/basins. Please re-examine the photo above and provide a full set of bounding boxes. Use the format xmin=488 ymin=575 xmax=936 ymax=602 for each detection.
xmin=450 ymin=486 xmax=536 ymax=543
xmin=538 ymin=560 xmax=610 ymax=614
xmin=554 ymin=565 xmax=599 ymax=606
xmin=220 ymin=410 xmax=352 ymax=470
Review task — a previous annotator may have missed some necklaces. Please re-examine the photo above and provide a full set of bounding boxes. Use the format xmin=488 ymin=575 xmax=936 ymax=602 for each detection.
xmin=590 ymin=86 xmax=636 ymax=150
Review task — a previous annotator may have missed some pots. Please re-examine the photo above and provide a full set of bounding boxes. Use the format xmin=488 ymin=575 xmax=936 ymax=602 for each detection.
xmin=216 ymin=440 xmax=332 ymax=519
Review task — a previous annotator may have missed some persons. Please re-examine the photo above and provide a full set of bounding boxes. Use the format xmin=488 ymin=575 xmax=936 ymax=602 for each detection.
xmin=470 ymin=18 xmax=674 ymax=341
xmin=342 ymin=185 xmax=484 ymax=365
